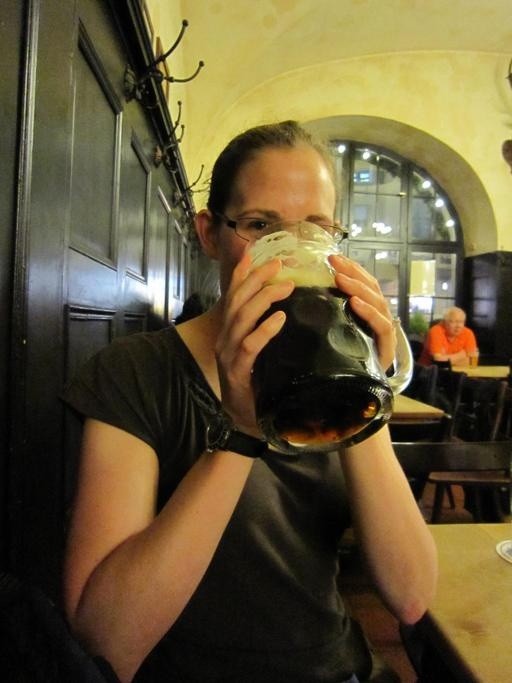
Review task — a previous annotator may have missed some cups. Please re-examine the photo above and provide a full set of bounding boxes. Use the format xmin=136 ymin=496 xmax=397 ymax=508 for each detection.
xmin=468 ymin=351 xmax=479 ymax=367
xmin=232 ymin=218 xmax=416 ymax=458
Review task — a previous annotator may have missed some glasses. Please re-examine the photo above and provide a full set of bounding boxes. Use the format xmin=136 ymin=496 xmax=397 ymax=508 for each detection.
xmin=218 ymin=213 xmax=349 ymax=246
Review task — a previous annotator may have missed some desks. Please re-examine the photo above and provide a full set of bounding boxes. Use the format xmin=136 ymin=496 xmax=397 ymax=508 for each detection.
xmin=397 ymin=519 xmax=511 ymax=683
xmin=385 ymin=391 xmax=446 ymax=428
xmin=449 ymin=356 xmax=511 ymax=411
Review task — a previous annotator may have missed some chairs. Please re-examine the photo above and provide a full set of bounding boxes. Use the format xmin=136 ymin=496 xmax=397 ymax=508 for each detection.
xmin=409 ymin=359 xmax=457 ymax=511
xmin=391 ymin=371 xmax=511 ymax=523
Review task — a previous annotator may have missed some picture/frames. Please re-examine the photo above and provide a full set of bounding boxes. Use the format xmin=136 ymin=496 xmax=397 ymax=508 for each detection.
xmin=155 ymin=36 xmax=170 ymax=102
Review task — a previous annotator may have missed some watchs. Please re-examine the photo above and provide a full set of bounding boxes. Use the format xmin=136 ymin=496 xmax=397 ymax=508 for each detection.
xmin=203 ymin=411 xmax=269 ymax=460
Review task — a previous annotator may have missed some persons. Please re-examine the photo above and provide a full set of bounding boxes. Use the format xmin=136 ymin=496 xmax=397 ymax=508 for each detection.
xmin=421 ymin=305 xmax=480 ymax=412
xmin=61 ymin=112 xmax=444 ymax=682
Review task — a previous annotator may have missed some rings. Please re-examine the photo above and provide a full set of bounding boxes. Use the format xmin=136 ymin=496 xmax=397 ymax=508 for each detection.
xmin=390 ymin=314 xmax=399 ymax=327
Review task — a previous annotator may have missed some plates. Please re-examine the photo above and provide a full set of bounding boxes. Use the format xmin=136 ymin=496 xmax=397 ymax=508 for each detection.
xmin=495 ymin=539 xmax=512 ymax=563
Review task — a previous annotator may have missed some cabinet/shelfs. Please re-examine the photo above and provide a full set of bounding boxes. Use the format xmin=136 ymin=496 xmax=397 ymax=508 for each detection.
xmin=458 ymin=248 xmax=512 ymax=363
